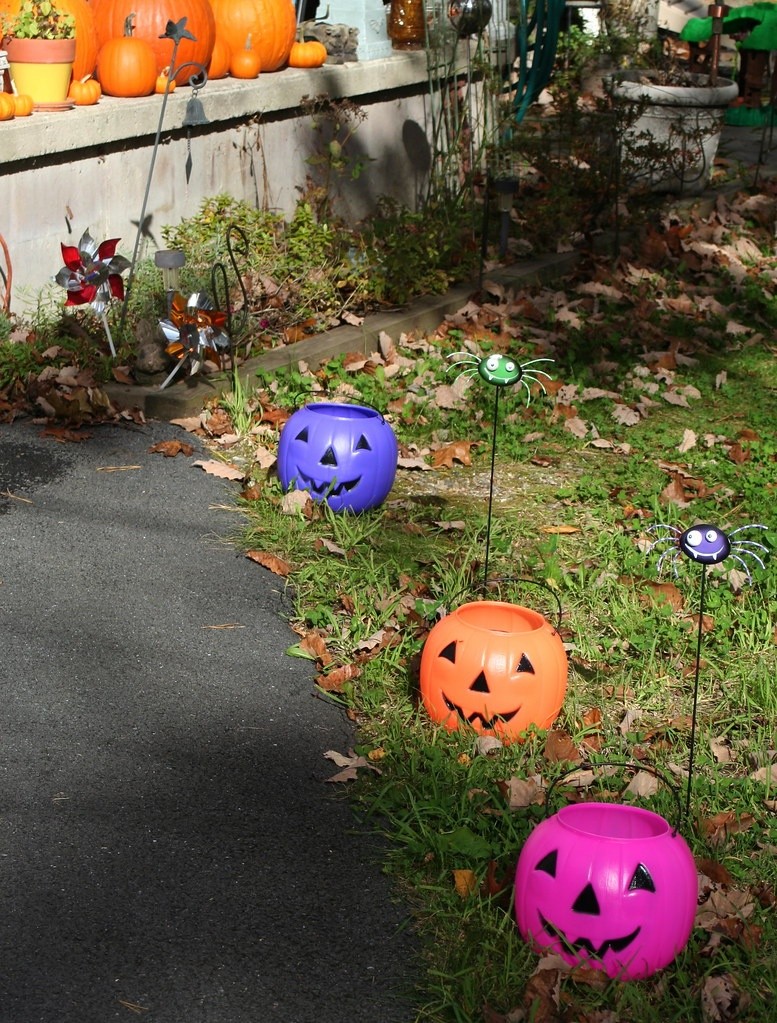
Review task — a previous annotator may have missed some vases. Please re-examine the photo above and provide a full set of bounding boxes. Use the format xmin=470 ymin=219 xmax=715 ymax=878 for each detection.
xmin=603 ymin=69 xmax=737 ymax=199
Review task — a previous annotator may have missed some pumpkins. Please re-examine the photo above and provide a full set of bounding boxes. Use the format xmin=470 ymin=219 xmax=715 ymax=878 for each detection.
xmin=0 ymin=0 xmax=327 ymax=121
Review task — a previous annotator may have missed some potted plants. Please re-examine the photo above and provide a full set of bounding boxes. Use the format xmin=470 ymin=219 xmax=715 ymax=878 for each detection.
xmin=1 ymin=1 xmax=76 ymax=104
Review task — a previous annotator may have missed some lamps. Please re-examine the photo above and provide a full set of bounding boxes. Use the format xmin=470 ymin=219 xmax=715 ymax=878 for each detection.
xmin=153 ymin=250 xmax=185 ymax=315
xmin=494 ymin=174 xmax=519 ymax=259
